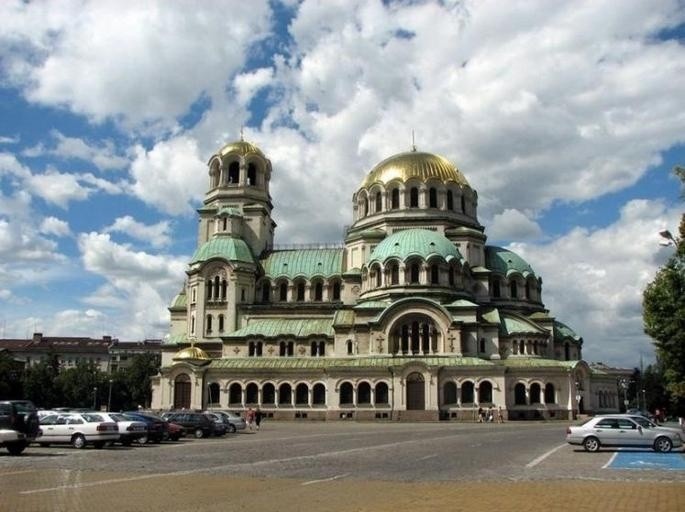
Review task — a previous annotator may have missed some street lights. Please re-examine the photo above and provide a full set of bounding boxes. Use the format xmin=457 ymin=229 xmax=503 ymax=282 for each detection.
xmin=618 ymin=375 xmax=634 ymax=411
xmin=573 ymin=374 xmax=583 ymax=416
xmin=107 ymin=378 xmax=114 ymax=412
xmin=93 ymin=387 xmax=98 ymax=407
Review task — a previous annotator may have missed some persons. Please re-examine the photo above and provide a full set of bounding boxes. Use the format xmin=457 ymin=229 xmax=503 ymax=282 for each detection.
xmin=245 ymin=406 xmax=255 ymax=431
xmin=677 ymin=414 xmax=685 ymax=426
xmin=477 ymin=407 xmax=485 ymax=423
xmin=487 ymin=405 xmax=494 ymax=422
xmin=579 ymin=396 xmax=585 ymax=413
xmin=653 ymin=408 xmax=665 ymax=423
xmin=254 ymin=404 xmax=262 ymax=429
xmin=497 ymin=405 xmax=505 ymax=424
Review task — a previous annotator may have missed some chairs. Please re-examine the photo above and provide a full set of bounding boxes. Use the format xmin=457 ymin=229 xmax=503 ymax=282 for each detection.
xmin=611 ymin=422 xmax=620 ymax=427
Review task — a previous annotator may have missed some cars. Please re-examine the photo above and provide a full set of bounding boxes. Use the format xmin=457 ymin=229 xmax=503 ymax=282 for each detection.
xmin=566 ymin=412 xmax=685 ymax=453
xmin=0 ymin=400 xmax=248 ymax=456
